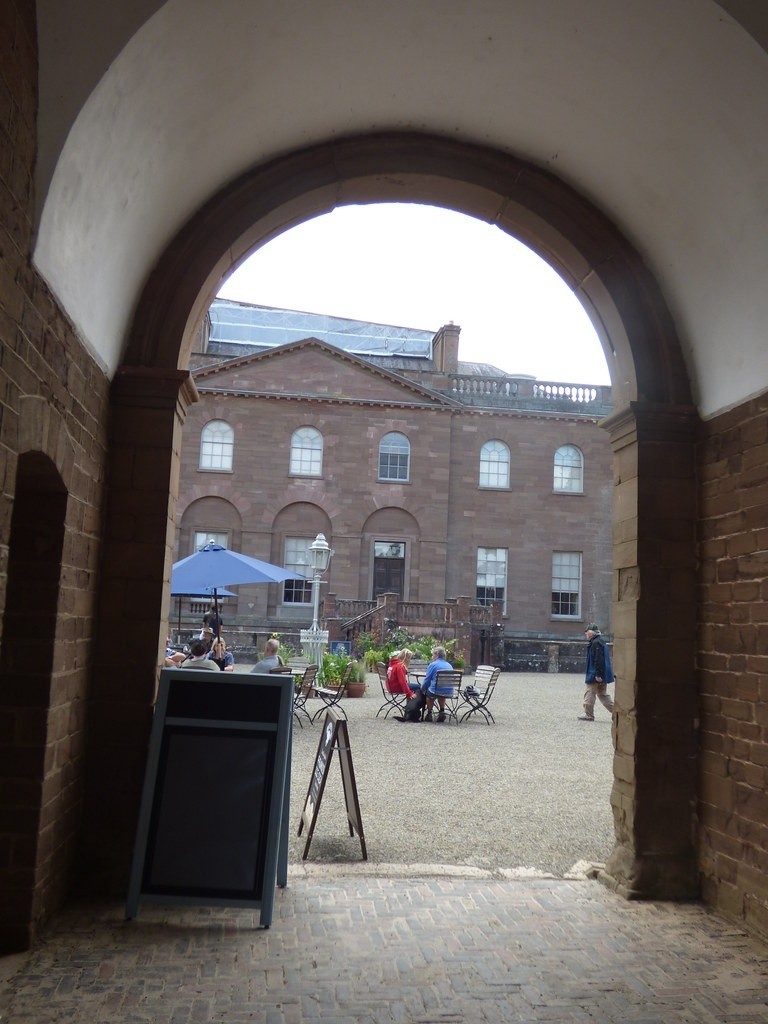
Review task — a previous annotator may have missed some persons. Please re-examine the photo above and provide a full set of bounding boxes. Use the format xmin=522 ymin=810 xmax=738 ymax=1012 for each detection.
xmin=164 ymin=627 xmax=234 ymax=672
xmin=248 ymin=639 xmax=283 ymax=674
xmin=386 ymin=648 xmax=420 ymax=703
xmin=201 ymin=602 xmax=224 ymax=633
xmin=419 ymin=647 xmax=454 ymax=723
xmin=578 ymin=623 xmax=614 ymax=722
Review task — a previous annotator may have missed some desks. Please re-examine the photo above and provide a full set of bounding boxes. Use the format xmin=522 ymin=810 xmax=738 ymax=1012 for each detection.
xmin=409 ymin=672 xmax=440 ymax=721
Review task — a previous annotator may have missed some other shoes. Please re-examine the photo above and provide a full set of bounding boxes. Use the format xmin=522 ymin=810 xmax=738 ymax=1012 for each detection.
xmin=425 ymin=713 xmax=432 ymax=722
xmin=577 ymin=714 xmax=595 ymax=721
xmin=436 ymin=711 xmax=446 ymax=723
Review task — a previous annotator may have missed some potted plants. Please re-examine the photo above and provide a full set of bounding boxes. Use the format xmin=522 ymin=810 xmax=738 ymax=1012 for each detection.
xmin=294 ymin=672 xmax=302 ymax=693
xmin=322 ymin=647 xmax=385 ymax=698
xmin=365 ymin=634 xmax=467 ymax=677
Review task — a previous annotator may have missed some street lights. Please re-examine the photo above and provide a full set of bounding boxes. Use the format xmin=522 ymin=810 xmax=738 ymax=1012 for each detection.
xmin=299 ymin=533 xmax=334 ymax=676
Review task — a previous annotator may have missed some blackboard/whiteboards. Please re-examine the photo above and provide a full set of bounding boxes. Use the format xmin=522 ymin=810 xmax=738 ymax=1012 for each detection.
xmin=302 ymin=710 xmax=365 ymax=841
xmin=127 ymin=667 xmax=296 ymax=908
xmin=331 ymin=641 xmax=352 ymax=655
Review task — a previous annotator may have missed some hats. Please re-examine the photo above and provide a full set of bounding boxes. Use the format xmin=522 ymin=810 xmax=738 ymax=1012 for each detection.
xmin=584 ymin=622 xmax=598 ymax=632
xmin=166 ymin=637 xmax=171 ymax=641
xmin=203 ymin=627 xmax=216 ymax=636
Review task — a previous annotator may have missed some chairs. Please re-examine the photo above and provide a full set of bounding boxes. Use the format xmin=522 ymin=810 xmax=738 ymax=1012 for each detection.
xmin=376 ymin=662 xmax=415 ymax=719
xmin=269 ymin=667 xmax=292 ymax=675
xmin=311 ymin=662 xmax=354 ymax=721
xmin=407 ymin=659 xmax=500 ymax=725
xmin=292 ymin=665 xmax=319 ymax=728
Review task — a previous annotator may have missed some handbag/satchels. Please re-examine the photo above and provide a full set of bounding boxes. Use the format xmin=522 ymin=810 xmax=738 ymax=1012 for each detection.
xmin=464 ymin=685 xmax=481 ymax=698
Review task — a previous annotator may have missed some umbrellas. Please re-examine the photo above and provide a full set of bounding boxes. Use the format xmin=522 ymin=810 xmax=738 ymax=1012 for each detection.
xmin=171 ymin=540 xmax=306 ymax=642
xmin=171 ymin=587 xmax=237 ymax=632
